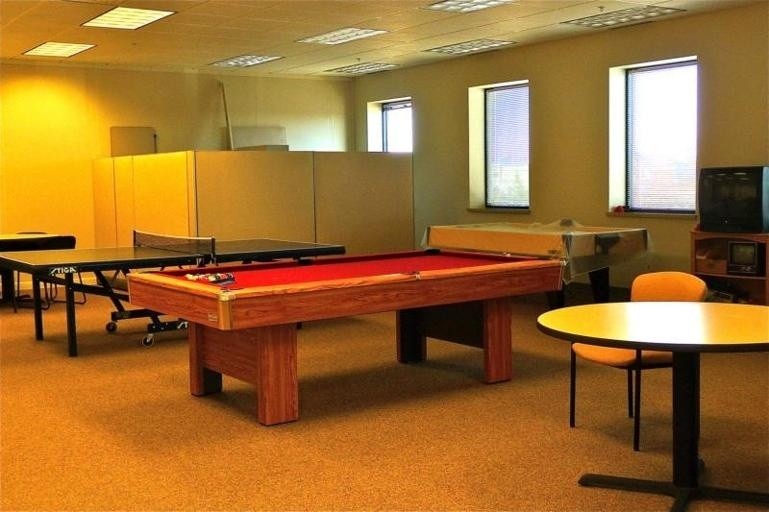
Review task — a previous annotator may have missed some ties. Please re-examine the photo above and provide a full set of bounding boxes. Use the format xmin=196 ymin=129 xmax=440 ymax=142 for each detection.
xmin=698 ymin=167 xmax=769 ymax=231
xmin=726 ymin=240 xmax=765 ymax=276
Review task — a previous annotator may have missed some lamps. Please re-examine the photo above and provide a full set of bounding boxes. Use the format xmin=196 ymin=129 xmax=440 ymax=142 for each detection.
xmin=569 ymin=271 xmax=707 ymax=450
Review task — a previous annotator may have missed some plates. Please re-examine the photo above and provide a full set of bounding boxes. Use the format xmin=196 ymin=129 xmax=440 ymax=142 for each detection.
xmin=690 ymin=228 xmax=769 ymax=306
